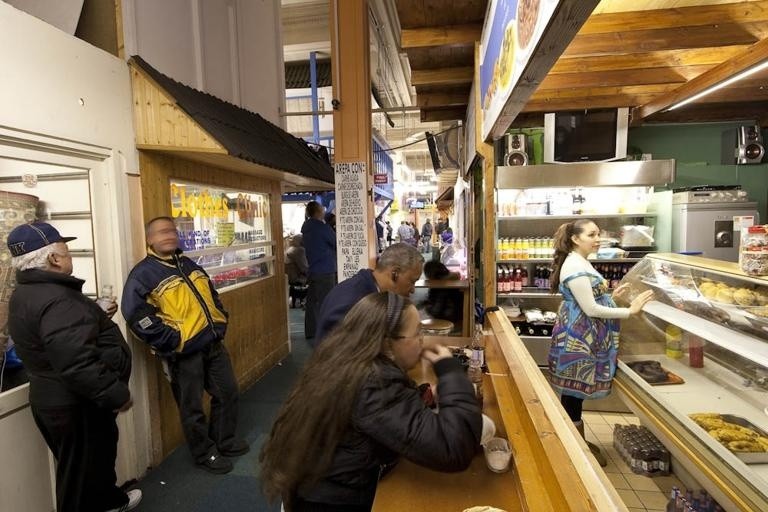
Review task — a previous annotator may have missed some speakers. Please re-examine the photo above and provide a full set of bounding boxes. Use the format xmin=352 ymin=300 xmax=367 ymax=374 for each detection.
xmin=498 ymin=132 xmax=529 ymax=166
xmin=721 ymin=125 xmax=765 ymax=165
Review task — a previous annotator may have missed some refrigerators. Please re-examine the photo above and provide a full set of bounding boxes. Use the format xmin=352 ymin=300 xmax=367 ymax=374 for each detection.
xmin=671 ymin=201 xmax=761 ymax=264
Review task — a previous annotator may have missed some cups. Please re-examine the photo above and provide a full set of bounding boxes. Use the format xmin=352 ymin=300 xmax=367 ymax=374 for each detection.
xmin=483 ymin=436 xmax=514 ymax=472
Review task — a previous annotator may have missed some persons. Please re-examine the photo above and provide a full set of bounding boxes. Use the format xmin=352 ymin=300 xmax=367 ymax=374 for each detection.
xmin=313 ymin=242 xmax=427 ymax=357
xmin=279 ymin=193 xmax=472 ymax=332
xmin=121 ymin=213 xmax=251 ymax=473
xmin=549 ymin=218 xmax=654 ymax=467
xmin=259 ymin=289 xmax=484 ymax=512
xmin=7 ymin=221 xmax=143 ymax=511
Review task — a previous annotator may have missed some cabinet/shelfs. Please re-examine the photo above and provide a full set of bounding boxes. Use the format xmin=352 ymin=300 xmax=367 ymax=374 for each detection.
xmin=609 ymin=253 xmax=766 ymax=512
xmin=493 ymin=157 xmax=676 ymax=336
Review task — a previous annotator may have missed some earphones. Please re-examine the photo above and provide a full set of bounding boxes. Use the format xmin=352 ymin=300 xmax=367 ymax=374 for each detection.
xmin=391 ymin=270 xmax=398 ymax=280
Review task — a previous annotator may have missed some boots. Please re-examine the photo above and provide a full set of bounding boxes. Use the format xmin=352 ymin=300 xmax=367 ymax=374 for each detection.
xmin=573 ymin=419 xmax=606 ymax=467
xmin=585 ymin=441 xmax=600 ymax=454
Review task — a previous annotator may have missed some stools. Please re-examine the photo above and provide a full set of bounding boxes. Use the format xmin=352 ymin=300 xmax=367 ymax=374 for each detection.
xmin=415 ymin=316 xmax=455 ymax=334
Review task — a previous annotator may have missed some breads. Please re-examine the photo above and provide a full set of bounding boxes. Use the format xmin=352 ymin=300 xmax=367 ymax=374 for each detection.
xmin=670 ymin=274 xmax=768 ymax=318
xmin=690 ymin=414 xmax=768 ymax=453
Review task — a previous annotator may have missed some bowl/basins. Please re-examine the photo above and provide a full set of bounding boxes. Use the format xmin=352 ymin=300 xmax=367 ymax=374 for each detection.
xmin=450 ymin=351 xmax=487 ymax=375
xmin=418 ymin=318 xmax=456 ymax=336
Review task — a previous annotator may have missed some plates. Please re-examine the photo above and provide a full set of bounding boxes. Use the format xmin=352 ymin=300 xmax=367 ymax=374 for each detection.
xmin=515 ymin=0 xmax=541 ymax=55
xmin=498 ymin=20 xmax=521 ymax=98
xmin=479 ymin=413 xmax=496 ymax=445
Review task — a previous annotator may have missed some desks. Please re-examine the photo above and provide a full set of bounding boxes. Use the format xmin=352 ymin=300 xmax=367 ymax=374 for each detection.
xmin=368 ymin=329 xmax=525 ymax=512
xmin=405 ymin=260 xmax=470 ymax=314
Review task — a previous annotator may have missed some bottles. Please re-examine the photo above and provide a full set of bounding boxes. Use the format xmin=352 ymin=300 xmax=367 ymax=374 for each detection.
xmin=612 ymin=423 xmax=672 ymax=478
xmin=666 ymin=486 xmax=727 ymax=512
xmin=97 ymin=284 xmax=113 ymax=320
xmin=496 ymin=235 xmax=557 ymax=259
xmin=498 ymin=263 xmax=634 ymax=292
xmin=473 ymin=323 xmax=486 ymax=368
xmin=661 ymin=324 xmax=705 ymax=370
xmin=468 ymin=359 xmax=482 ymax=404
xmin=512 ymin=323 xmax=552 ymax=337
xmin=740 ymin=224 xmax=768 ymax=277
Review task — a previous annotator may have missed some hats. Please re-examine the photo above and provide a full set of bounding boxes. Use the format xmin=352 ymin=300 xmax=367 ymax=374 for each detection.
xmin=7 ymin=222 xmax=77 ymax=257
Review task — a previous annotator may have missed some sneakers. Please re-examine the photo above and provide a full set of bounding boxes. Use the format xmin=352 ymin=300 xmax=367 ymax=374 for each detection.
xmin=216 ymin=439 xmax=249 ymax=457
xmin=194 ymin=455 xmax=233 ymax=474
xmin=104 ymin=488 xmax=143 ymax=512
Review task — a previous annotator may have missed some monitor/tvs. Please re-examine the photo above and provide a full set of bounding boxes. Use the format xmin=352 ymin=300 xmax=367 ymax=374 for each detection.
xmin=543 ymin=106 xmax=629 ymax=165
xmin=424 ymin=131 xmax=441 ymax=175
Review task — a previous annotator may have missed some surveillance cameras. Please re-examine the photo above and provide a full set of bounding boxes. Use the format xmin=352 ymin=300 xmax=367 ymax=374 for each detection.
xmin=332 ymin=99 xmax=340 ymax=111
